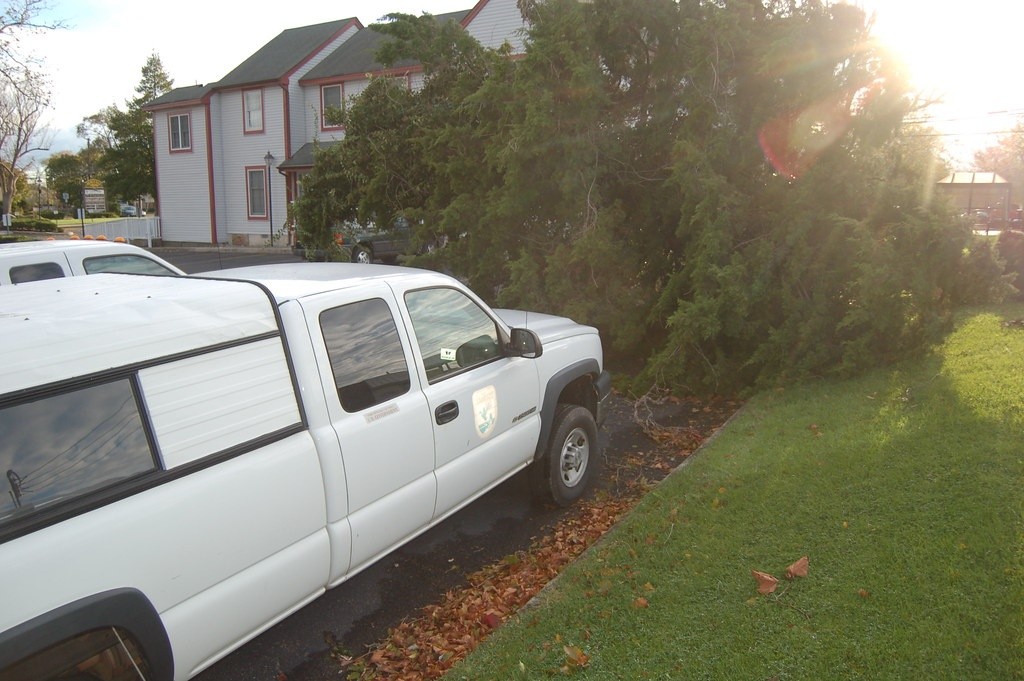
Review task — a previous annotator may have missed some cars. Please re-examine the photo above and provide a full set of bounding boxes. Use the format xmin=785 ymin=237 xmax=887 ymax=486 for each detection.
xmin=307 ymin=205 xmax=442 ymax=265
xmin=120 ymin=205 xmax=147 ymax=218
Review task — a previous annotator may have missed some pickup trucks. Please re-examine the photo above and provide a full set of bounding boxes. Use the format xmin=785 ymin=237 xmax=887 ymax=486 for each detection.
xmin=1 ymin=256 xmax=615 ymax=681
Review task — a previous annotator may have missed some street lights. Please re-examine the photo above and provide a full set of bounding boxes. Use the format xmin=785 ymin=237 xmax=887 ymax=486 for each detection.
xmin=263 ymin=150 xmax=275 ymax=245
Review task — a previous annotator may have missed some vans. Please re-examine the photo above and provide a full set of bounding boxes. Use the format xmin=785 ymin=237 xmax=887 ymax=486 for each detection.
xmin=1 ymin=238 xmax=187 ymax=282
